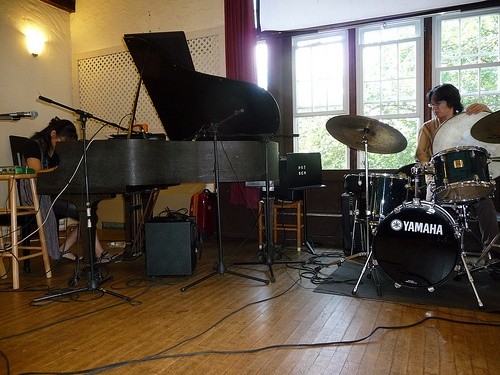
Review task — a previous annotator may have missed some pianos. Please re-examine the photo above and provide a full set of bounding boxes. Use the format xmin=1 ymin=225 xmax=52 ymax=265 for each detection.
xmin=36 ymin=31 xmax=281 ymax=278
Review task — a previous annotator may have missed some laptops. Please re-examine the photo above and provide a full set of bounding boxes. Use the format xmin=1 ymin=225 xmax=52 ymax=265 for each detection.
xmin=278 ymin=151 xmax=320 ymax=188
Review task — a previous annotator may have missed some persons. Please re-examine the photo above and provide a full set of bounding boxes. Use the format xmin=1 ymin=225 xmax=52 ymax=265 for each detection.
xmin=416 ymin=84 xmax=500 ymax=270
xmin=19 ymin=116 xmax=121 ymax=264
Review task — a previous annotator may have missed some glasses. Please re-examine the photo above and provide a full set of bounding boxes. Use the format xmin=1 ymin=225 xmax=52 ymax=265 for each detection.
xmin=427 ymin=101 xmax=447 ymax=108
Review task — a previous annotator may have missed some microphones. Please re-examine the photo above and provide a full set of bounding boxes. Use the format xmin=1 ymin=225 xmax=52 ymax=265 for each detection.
xmin=139 ymin=126 xmax=148 ymax=140
xmin=9 ymin=111 xmax=38 ymax=119
xmin=192 ymin=124 xmax=205 ymax=142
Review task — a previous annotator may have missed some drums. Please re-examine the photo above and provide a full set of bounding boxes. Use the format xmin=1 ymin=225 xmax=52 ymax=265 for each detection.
xmin=372 ymin=200 xmax=461 ymax=291
xmin=355 ymin=173 xmax=411 ymax=226
xmin=413 ymin=165 xmax=426 ymax=200
xmin=432 ymin=111 xmax=500 ymax=180
xmin=430 ymin=146 xmax=496 ymax=203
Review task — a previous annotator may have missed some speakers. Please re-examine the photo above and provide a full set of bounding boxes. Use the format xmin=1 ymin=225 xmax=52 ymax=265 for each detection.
xmin=144 ymin=217 xmax=196 ymax=277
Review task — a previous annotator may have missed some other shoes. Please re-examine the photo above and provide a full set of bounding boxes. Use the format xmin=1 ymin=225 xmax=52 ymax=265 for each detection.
xmin=486 ymin=252 xmax=499 ymax=266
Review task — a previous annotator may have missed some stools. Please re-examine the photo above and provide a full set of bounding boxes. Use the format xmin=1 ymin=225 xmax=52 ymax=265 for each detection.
xmin=258 ymin=197 xmax=305 ymax=251
xmin=0 ymin=174 xmax=52 ymax=290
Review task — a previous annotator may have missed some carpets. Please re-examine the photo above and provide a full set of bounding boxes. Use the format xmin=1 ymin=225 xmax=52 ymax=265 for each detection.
xmin=311 ymin=256 xmax=500 ymax=315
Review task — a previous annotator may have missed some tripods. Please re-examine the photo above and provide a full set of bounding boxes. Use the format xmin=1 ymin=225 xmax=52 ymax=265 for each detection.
xmin=315 ymin=128 xmax=383 ymax=295
xmin=180 ymin=109 xmax=306 ymax=291
xmin=32 ymin=96 xmax=139 ymax=302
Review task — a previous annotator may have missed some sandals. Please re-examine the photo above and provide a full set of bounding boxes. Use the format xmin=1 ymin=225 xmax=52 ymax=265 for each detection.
xmin=60 ymin=250 xmax=83 ymax=264
xmin=96 ymin=251 xmax=119 ymax=262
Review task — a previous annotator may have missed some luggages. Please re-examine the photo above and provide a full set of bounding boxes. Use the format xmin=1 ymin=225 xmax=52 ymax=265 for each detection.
xmin=190 ymin=189 xmax=217 ymax=239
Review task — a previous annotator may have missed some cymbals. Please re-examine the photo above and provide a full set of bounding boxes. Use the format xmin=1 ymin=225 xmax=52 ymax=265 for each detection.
xmin=471 ymin=110 xmax=500 ymax=144
xmin=326 ymin=115 xmax=408 ymax=154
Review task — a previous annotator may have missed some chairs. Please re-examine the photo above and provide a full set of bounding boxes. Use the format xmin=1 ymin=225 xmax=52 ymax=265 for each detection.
xmin=8 ymin=135 xmax=112 ymax=273
